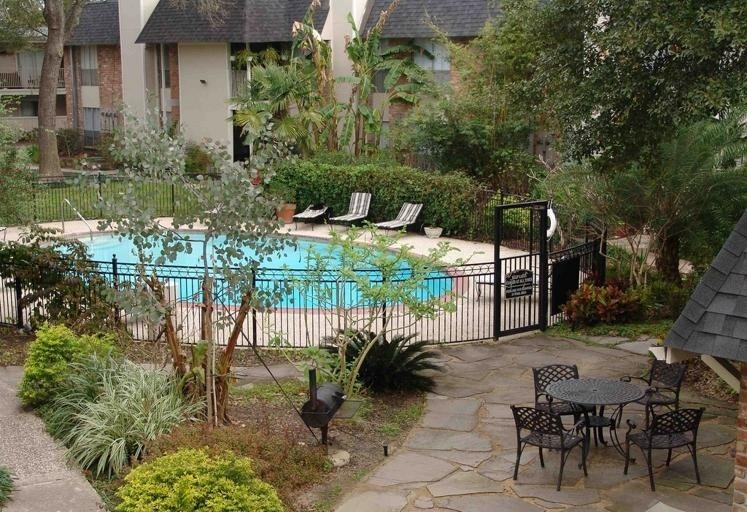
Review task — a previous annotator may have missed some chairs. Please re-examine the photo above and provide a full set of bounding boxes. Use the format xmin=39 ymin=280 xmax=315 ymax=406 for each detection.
xmin=477 ymin=267 xmax=553 ymax=303
xmin=623 ymin=407 xmax=706 ymax=491
xmin=617 ymin=358 xmax=688 ymax=428
xmin=291 ymin=204 xmax=329 ymax=231
xmin=531 ymin=363 xmax=598 ymax=453
xmin=371 ymin=202 xmax=423 ymax=240
xmin=329 ymin=192 xmax=372 ymax=235
xmin=509 ymin=404 xmax=589 ymax=491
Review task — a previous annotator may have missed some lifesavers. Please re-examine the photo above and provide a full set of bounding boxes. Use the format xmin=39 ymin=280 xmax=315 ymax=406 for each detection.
xmin=547 ymin=209 xmax=556 ymax=238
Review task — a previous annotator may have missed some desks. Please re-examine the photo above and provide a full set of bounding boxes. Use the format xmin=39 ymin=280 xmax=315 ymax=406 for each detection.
xmin=545 ymin=378 xmax=646 ymax=470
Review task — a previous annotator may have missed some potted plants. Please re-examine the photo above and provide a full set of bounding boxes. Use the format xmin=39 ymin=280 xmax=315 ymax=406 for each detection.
xmin=424 ymin=218 xmax=443 ymax=239
xmin=269 ymin=182 xmax=297 ymax=224
